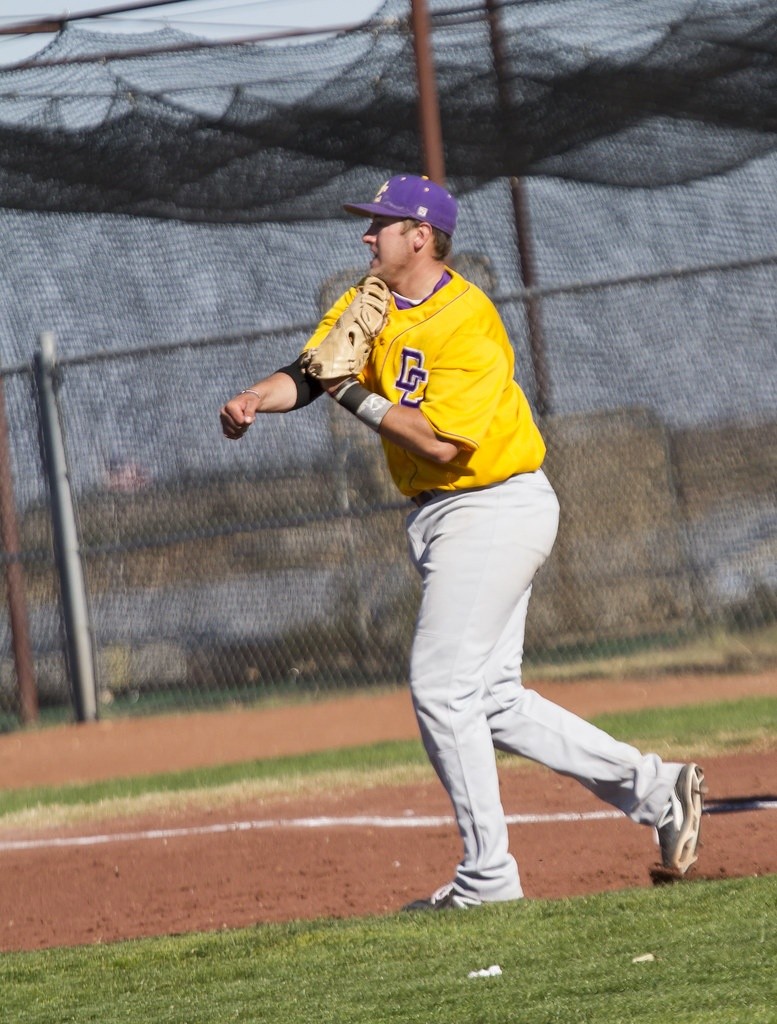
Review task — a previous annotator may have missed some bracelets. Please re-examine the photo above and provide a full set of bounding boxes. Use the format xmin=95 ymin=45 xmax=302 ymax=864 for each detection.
xmin=238 ymin=390 xmax=261 ymax=399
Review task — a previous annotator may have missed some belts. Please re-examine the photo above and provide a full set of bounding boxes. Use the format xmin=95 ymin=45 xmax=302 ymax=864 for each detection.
xmin=410 ymin=489 xmax=442 ymax=508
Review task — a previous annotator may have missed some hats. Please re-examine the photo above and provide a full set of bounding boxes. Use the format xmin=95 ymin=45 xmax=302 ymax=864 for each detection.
xmin=342 ymin=176 xmax=458 ymax=236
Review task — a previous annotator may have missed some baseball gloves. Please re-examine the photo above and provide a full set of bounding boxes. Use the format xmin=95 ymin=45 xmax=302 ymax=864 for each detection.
xmin=305 ymin=275 xmax=392 ymax=380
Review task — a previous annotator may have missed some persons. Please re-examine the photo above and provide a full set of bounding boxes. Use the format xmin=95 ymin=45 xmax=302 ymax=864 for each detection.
xmin=219 ymin=176 xmax=704 ymax=914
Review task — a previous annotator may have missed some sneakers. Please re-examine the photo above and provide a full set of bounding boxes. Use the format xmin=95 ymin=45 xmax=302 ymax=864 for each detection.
xmin=400 ymin=899 xmax=461 ymax=919
xmin=649 ymin=764 xmax=705 ymax=875
